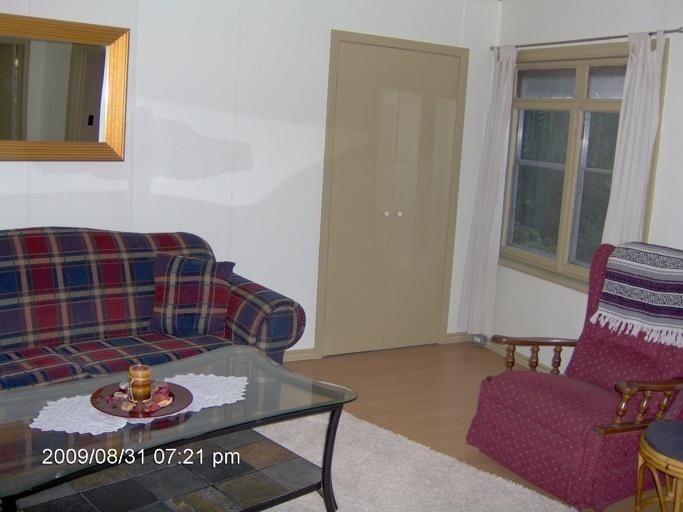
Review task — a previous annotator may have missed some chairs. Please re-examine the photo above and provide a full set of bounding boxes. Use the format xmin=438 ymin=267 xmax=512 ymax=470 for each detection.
xmin=465 ymin=241 xmax=683 ymax=512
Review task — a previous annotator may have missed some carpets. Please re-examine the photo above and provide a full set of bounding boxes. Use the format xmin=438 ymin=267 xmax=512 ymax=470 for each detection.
xmin=248 ymin=408 xmax=579 ymax=512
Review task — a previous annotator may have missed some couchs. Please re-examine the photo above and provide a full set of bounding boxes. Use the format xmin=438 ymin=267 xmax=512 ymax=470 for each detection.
xmin=0 ymin=226 xmax=306 ymax=479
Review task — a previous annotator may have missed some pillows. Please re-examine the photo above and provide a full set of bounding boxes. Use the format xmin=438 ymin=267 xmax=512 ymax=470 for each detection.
xmin=147 ymin=252 xmax=236 ymax=338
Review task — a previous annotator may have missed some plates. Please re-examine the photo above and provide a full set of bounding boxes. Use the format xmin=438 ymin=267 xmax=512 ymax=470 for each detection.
xmin=90 ymin=378 xmax=193 ymax=418
